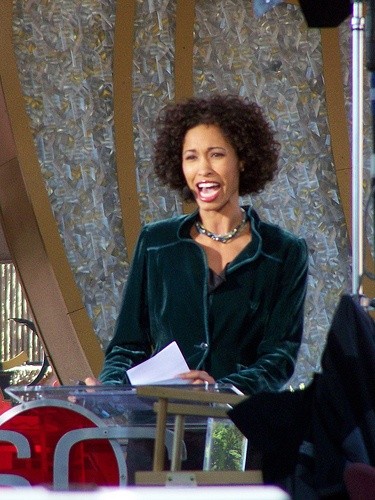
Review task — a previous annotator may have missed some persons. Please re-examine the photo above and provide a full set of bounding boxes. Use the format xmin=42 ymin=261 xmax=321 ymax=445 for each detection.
xmin=65 ymin=94 xmax=310 ymax=487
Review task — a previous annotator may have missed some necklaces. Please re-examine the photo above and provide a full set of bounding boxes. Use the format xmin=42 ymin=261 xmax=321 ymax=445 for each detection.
xmin=194 ymin=205 xmax=247 ymax=242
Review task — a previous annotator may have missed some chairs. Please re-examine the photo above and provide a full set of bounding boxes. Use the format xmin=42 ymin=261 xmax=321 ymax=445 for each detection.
xmin=134 ymin=293 xmax=375 ymax=500
